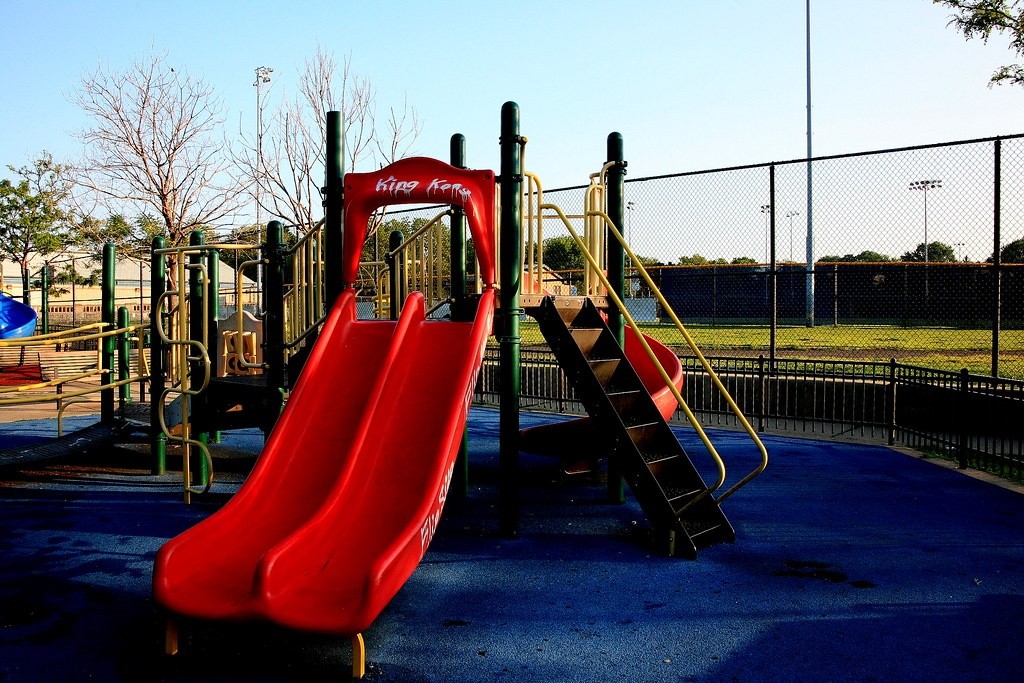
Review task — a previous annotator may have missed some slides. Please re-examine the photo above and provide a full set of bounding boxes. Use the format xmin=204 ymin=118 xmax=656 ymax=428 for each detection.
xmin=0 ymin=291 xmax=38 ymax=340
xmin=572 ymin=308 xmax=686 ymax=474
xmin=151 ymin=286 xmax=502 ymax=635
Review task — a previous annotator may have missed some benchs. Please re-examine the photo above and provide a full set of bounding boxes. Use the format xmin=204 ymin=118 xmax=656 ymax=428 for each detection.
xmin=37 ymin=347 xmax=154 ymax=409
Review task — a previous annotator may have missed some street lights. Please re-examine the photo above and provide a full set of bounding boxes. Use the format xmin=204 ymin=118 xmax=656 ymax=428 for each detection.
xmin=627 ymin=202 xmax=634 ymax=297
xmin=909 ymin=180 xmax=943 ymax=304
xmin=787 ymin=210 xmax=799 ymax=290
xmin=954 ymin=244 xmax=965 ymax=262
xmin=253 ymin=65 xmax=274 ymax=320
xmin=761 ymin=205 xmax=770 ymax=306
